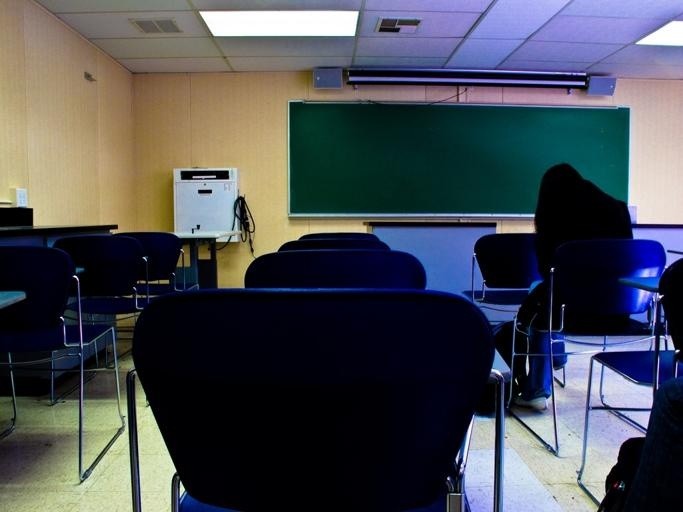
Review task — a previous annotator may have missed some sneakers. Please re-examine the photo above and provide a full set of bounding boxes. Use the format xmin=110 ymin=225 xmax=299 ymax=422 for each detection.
xmin=514 ymin=397 xmax=548 ymax=410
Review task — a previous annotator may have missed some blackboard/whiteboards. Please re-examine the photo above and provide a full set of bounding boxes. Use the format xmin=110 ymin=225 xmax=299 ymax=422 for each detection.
xmin=287 ymin=100 xmax=631 ymax=219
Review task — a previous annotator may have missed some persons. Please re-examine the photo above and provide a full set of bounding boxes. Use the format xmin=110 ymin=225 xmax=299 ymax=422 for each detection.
xmin=496 ymin=162 xmax=636 ymax=411
xmin=596 ymin=258 xmax=683 ymax=512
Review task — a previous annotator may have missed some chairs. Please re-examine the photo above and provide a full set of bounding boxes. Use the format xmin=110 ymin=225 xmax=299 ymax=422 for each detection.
xmin=278 ymin=240 xmax=389 ymax=250
xmin=0 ymin=199 xmax=200 ymax=483
xmin=465 ymin=230 xmax=683 ymax=512
xmin=299 ymin=231 xmax=378 ymax=240
xmin=245 ymin=250 xmax=427 ymax=289
xmin=128 ymin=290 xmax=507 ymax=512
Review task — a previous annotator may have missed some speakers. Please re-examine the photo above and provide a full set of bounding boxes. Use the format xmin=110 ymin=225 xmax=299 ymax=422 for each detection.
xmin=586 ymin=75 xmax=617 ymax=96
xmin=312 ymin=68 xmax=344 ymax=90
